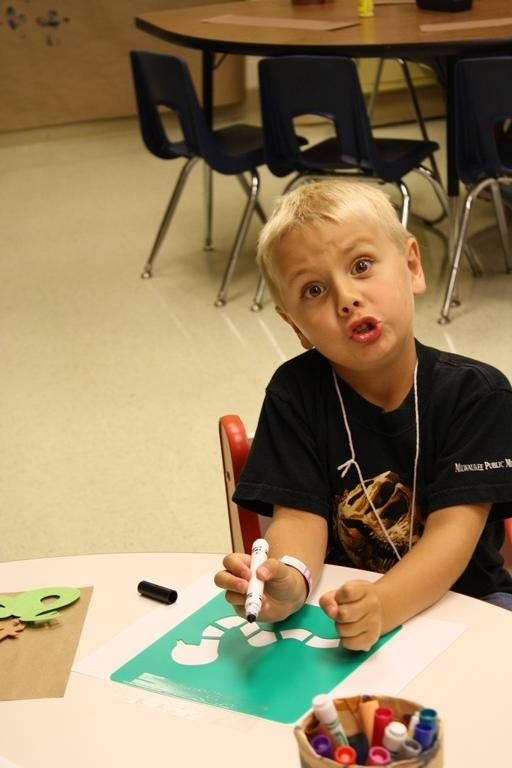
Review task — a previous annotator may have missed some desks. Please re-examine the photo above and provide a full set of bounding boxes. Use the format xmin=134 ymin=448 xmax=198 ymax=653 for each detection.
xmin=1 ymin=552 xmax=512 ymax=768
xmin=134 ymin=1 xmax=511 ymax=252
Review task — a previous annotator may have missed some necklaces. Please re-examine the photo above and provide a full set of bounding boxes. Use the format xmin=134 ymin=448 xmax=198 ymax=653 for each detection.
xmin=329 ymin=367 xmax=418 ymax=560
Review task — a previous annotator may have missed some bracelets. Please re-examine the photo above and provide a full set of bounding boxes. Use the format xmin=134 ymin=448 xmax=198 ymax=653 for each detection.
xmin=280 ymin=553 xmax=314 ymax=606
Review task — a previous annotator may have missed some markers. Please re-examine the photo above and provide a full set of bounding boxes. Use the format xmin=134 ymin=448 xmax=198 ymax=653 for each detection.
xmin=244 ymin=537 xmax=270 ymax=624
xmin=304 ymin=692 xmax=438 ymax=767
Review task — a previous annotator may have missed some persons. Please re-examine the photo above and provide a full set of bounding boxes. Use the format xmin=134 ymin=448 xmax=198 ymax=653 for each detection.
xmin=214 ymin=180 xmax=512 ymax=651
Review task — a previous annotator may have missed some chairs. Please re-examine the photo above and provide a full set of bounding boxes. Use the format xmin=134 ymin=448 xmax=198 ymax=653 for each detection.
xmin=436 ymin=57 xmax=512 ymax=324
xmin=129 ymin=50 xmax=310 ymax=306
xmin=218 ymin=414 xmax=512 ymax=578
xmin=250 ymin=55 xmax=481 ymax=312
